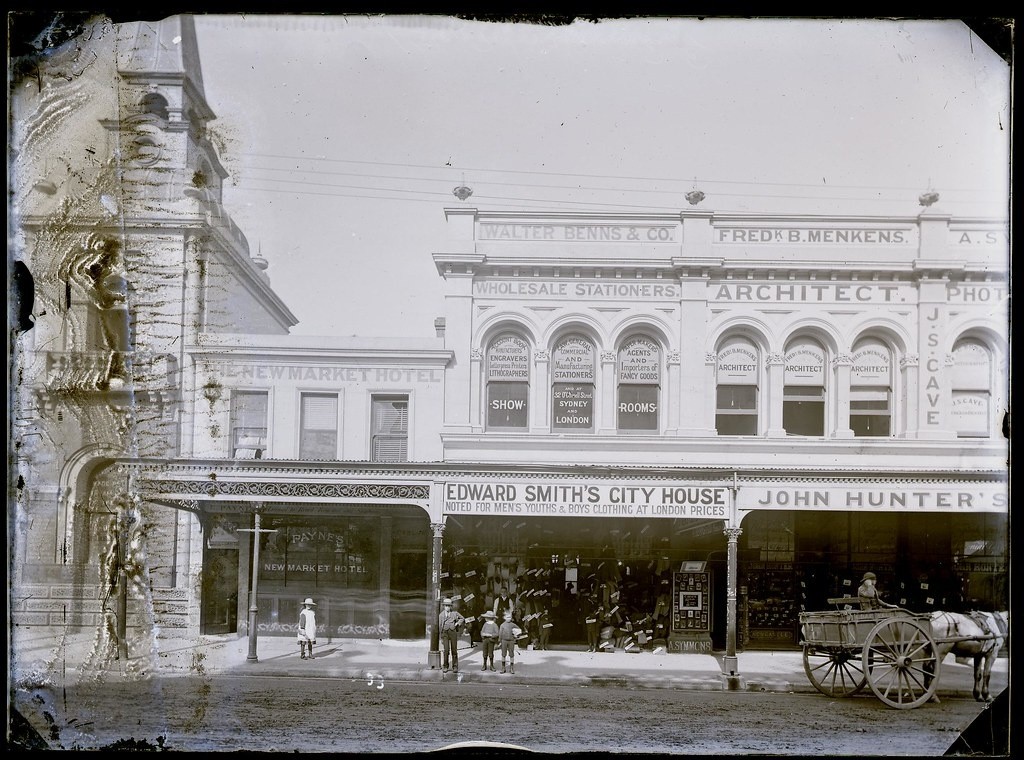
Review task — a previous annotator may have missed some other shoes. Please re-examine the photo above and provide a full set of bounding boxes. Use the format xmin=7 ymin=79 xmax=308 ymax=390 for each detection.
xmin=453 ymin=667 xmax=458 ymax=672
xmin=309 ymin=654 xmax=315 ymax=658
xmin=443 ymin=668 xmax=448 ymax=672
xmin=301 ymin=655 xmax=307 ymax=658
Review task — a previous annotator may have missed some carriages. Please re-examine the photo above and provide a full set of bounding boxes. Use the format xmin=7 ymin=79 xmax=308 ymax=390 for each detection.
xmin=798 ymin=593 xmax=1009 ymax=710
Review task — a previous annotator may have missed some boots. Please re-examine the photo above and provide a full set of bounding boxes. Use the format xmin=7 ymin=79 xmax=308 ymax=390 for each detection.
xmin=481 ymin=659 xmax=486 ymax=671
xmin=500 ymin=662 xmax=506 ymax=673
xmin=510 ymin=664 xmax=514 ymax=673
xmin=490 ymin=658 xmax=496 ymax=671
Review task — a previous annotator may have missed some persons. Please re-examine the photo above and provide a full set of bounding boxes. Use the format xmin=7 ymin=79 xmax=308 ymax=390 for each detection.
xmin=857 ymin=572 xmax=900 ymax=613
xmin=438 ymin=598 xmax=465 ymax=674
xmin=480 ymin=586 xmax=523 ymax=675
xmin=538 ymin=608 xmax=553 ymax=651
xmin=585 ymin=611 xmax=601 ymax=653
xmin=294 ymin=596 xmax=318 ymax=660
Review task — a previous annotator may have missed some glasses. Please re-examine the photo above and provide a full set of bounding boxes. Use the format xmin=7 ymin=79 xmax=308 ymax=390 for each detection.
xmin=445 ymin=605 xmax=450 ymax=607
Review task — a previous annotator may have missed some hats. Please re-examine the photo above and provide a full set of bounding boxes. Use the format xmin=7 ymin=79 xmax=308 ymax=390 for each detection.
xmin=300 ymin=598 xmax=318 ymax=605
xmin=860 ymin=572 xmax=876 ymax=582
xmin=481 ymin=611 xmax=497 ymax=617
xmin=441 ymin=599 xmax=453 ymax=605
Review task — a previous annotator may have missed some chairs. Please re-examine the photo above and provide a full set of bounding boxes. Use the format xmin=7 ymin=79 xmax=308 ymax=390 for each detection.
xmin=827 ymin=598 xmax=873 ymax=610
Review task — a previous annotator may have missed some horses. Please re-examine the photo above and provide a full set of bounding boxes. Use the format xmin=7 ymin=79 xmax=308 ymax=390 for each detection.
xmin=922 ymin=610 xmax=1009 ymax=704
